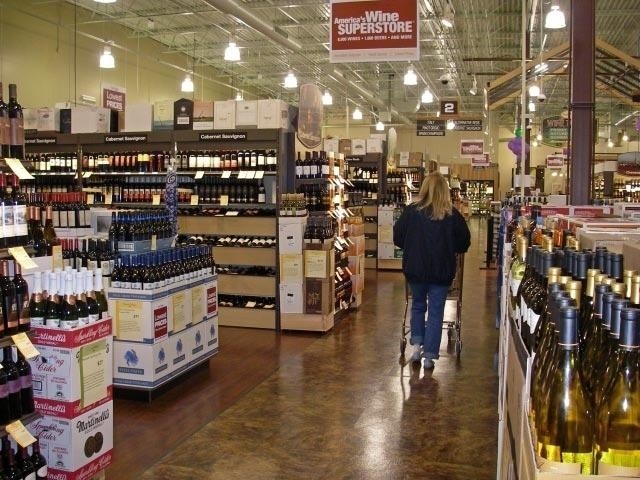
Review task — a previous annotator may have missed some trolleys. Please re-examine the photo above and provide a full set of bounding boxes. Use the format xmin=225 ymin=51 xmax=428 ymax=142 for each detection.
xmin=399 ymin=248 xmax=463 ymax=361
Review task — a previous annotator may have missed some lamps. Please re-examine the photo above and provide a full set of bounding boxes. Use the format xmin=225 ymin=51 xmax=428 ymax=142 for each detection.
xmin=181 ymin=76 xmax=195 ymax=93
xmin=545 ymin=0 xmax=567 ymax=29
xmin=284 ymin=69 xmax=298 ymax=88
xmin=322 ymin=89 xmax=332 ymax=105
xmin=98 ymin=45 xmax=116 ymax=69
xmin=352 ymin=106 xmax=362 ymax=120
xmin=403 ymin=67 xmax=418 ymax=86
xmin=224 ymin=38 xmax=241 ymax=61
xmin=376 ymin=122 xmax=385 ymax=131
xmin=422 ymin=89 xmax=434 ymax=103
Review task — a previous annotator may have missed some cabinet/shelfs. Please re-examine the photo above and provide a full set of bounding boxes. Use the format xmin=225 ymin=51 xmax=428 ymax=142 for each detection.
xmin=464 ymin=180 xmax=495 ymax=216
xmin=20 ymin=130 xmax=296 ymax=331
xmin=347 ymin=153 xmax=387 ymax=268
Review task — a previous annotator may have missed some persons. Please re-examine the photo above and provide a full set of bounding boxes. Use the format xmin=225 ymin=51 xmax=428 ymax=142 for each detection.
xmin=393 ymin=171 xmax=471 ymax=369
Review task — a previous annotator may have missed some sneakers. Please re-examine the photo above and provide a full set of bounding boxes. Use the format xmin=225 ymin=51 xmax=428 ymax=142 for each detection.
xmin=424 ymin=359 xmax=435 ymax=369
xmin=411 ymin=352 xmax=420 ymax=361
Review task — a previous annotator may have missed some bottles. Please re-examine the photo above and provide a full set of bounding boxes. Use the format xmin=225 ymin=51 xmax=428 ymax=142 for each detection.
xmin=295 ymin=152 xmax=303 ymax=178
xmin=244 ymin=147 xmax=251 ymax=170
xmin=595 ymin=179 xmax=639 ymax=202
xmin=250 ymin=148 xmax=258 ymax=170
xmin=27 ymin=178 xmax=111 ymax=203
xmin=189 ymin=150 xmax=197 ymax=170
xmin=164 ymin=154 xmax=169 ymax=171
xmin=302 ymin=184 xmax=311 ymax=210
xmin=131 ymin=150 xmax=137 ymax=173
xmin=231 ymin=150 xmax=239 ymax=172
xmin=58 ymin=273 xmax=79 ymax=331
xmin=58 ymin=203 xmax=91 ymax=229
xmin=96 ymin=152 xmax=103 ymax=171
xmin=12 ymin=260 xmax=30 ymax=329
xmin=110 ymin=254 xmax=121 ymax=286
xmin=75 ymin=272 xmax=88 ymax=326
xmin=265 ymin=147 xmax=273 ymax=172
xmin=13 ymin=439 xmax=35 ymax=480
xmin=115 ymin=152 xmax=120 ymax=174
xmin=302 ymin=151 xmax=311 ymax=178
xmin=152 ymin=253 xmax=161 ymax=288
xmin=0 ymin=298 xmax=6 ymax=337
xmin=50 ymin=155 xmax=56 ymax=172
xmin=258 ymin=149 xmax=266 ymax=170
xmin=107 ymin=151 xmax=114 ymax=174
xmin=188 ymin=234 xmax=197 ymax=244
xmin=61 ymin=239 xmax=70 ymax=271
xmin=1 ymin=259 xmax=20 ymax=334
xmin=125 ymin=151 xmax=132 ymax=173
xmin=150 ymin=154 xmax=156 ymax=172
xmin=215 ymin=298 xmax=275 ymax=310
xmin=31 ymin=434 xmax=47 ymax=480
xmin=262 ymin=236 xmax=276 ymax=248
xmin=43 ymin=271 xmax=60 ymax=329
xmin=225 ymin=151 xmax=232 ymax=170
xmin=111 ymin=177 xmax=120 ymax=202
xmin=237 ymin=149 xmax=245 ymax=171
xmin=142 ymin=257 xmax=155 ymax=289
xmin=257 ymin=183 xmax=266 ymax=204
xmin=100 ymin=242 xmax=111 ymax=276
xmin=310 ymin=184 xmax=318 ymax=212
xmin=216 ymin=267 xmax=225 ymax=273
xmin=82 ymin=269 xmax=101 ymax=321
xmin=174 ymin=150 xmax=182 ymax=170
xmin=198 ymin=183 xmax=205 ymax=203
xmin=319 ymin=184 xmax=327 ymax=211
xmin=203 ymin=150 xmax=210 ymax=170
xmin=30 ymin=207 xmax=47 ymax=257
xmin=217 ymin=238 xmax=226 ymax=245
xmin=120 ymin=152 xmax=126 ymax=174
xmin=349 ymin=167 xmax=379 ymax=178
xmin=60 ymin=153 xmax=67 ymax=172
xmin=388 ymin=186 xmax=409 ymax=201
xmin=251 ymin=236 xmax=260 ymax=247
xmin=89 ymin=239 xmax=99 ymax=276
xmin=108 ymin=212 xmax=120 ymax=257
xmin=161 ymin=175 xmax=166 ymax=203
xmin=157 ymin=177 xmax=162 ymax=196
xmin=210 ymin=185 xmax=217 ymax=203
xmin=509 ymin=224 xmax=640 ymax=474
xmin=257 ymin=235 xmax=267 ymax=246
xmin=328 ymin=186 xmax=331 ymax=210
xmin=222 ymin=264 xmax=234 ymax=275
xmin=43 ymin=203 xmax=57 ymax=243
xmin=221 ymin=236 xmax=233 ymax=247
xmin=54 ymin=154 xmax=62 ymax=173
xmin=0 ymin=447 xmax=23 ymax=479
xmin=150 ymin=175 xmax=157 ymax=200
xmin=195 ymin=233 xmax=206 ymax=245
xmin=346 ymin=189 xmax=372 ymax=207
xmin=138 ymin=151 xmax=144 ymax=172
xmin=181 ymin=149 xmax=189 ymax=170
xmin=132 ymin=177 xmax=139 ymax=203
xmin=178 ymin=187 xmax=195 ymax=204
xmin=28 ymin=269 xmax=45 ymax=327
xmin=209 ymin=150 xmax=214 ymax=170
xmin=65 ymin=151 xmax=73 ymax=173
xmin=145 ymin=175 xmax=151 ymax=203
xmin=335 ymin=245 xmax=353 ymax=265
xmin=310 ymin=151 xmax=319 ymax=178
xmin=387 ymin=170 xmax=420 ymax=183
xmin=196 ymin=150 xmax=203 ymax=169
xmin=77 ymin=239 xmax=88 ymax=269
xmin=139 ymin=175 xmax=145 ymax=202
xmin=239 ymin=236 xmax=253 ymax=246
xmin=16 ymin=342 xmax=35 ymax=414
xmin=101 ymin=152 xmax=107 ymax=173
xmin=11 ymin=170 xmax=26 ymax=224
xmin=206 ymin=235 xmax=219 ymax=245
xmin=242 ymin=185 xmax=249 ymax=205
xmin=123 ymin=177 xmax=129 ymax=203
xmin=304 ymin=216 xmax=335 ymax=243
xmin=88 ymin=154 xmax=94 ymax=170
xmin=1 ymin=82 xmax=11 ymax=158
xmin=214 ymin=150 xmax=221 ymax=171
xmin=157 ymin=153 xmax=164 ymax=172
xmin=2 ymin=346 xmax=24 ymax=420
xmin=1 ymin=347 xmax=10 ymax=425
xmin=128 ymin=177 xmax=134 ymax=202
xmin=1 ymin=173 xmax=15 ymax=224
xmin=229 ymin=185 xmax=236 ymax=203
xmin=235 ymin=235 xmax=248 ymax=248
xmin=317 ymin=151 xmax=326 ymax=177
xmin=126 ymin=212 xmax=135 ymax=243
xmin=236 ymin=185 xmax=242 ymax=203
xmin=248 ymin=184 xmax=256 ymax=204
xmin=119 ymin=254 xmax=131 ymax=288
xmin=205 ymin=185 xmax=211 ymax=203
xmin=40 ymin=155 xmax=45 ymax=172
xmin=131 ymin=255 xmax=142 ymax=289
xmin=143 ymin=152 xmax=150 ymax=173
xmin=230 ymin=237 xmax=238 ymax=247
xmin=45 ymin=155 xmax=50 ymax=171
xmin=117 ymin=213 xmax=127 ymax=242
xmin=70 ymin=152 xmax=78 ymax=171
xmin=6 ymin=83 xmax=23 ymax=159
xmin=89 ymin=267 xmax=108 ymax=318
xmin=220 ymin=152 xmax=225 ymax=171
xmin=216 ymin=186 xmax=223 ymax=203
